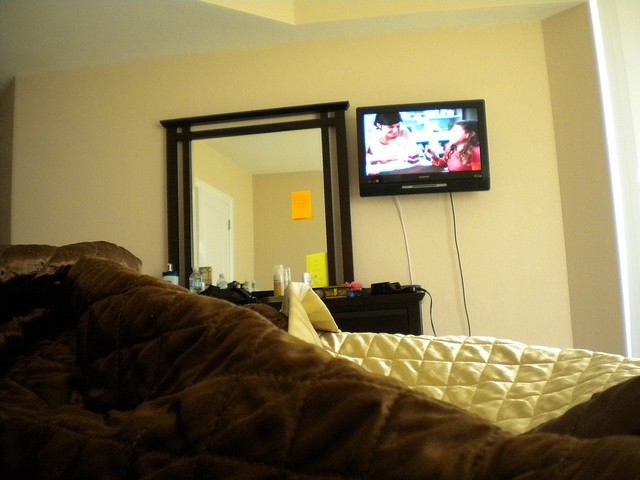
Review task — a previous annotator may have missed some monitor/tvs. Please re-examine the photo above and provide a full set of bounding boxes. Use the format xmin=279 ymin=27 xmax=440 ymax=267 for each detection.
xmin=356 ymin=99 xmax=491 ymax=198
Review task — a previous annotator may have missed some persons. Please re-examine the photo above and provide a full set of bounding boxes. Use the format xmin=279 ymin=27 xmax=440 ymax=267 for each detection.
xmin=425 ymin=120 xmax=481 ymax=173
xmin=364 ymin=112 xmax=424 ymax=165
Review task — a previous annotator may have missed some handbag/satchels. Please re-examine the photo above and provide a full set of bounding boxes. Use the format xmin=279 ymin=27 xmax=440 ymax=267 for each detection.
xmin=199 ymin=285 xmax=257 ymax=305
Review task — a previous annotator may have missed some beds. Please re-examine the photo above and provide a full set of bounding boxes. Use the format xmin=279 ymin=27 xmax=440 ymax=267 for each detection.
xmin=0 ymin=240 xmax=640 ymax=478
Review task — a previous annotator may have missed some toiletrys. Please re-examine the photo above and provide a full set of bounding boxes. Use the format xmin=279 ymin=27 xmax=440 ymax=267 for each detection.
xmin=273 ymin=265 xmax=284 ymax=297
xmin=217 ymin=273 xmax=257 ymax=294
xmin=302 ymin=272 xmax=312 ymax=289
xmin=283 ymin=267 xmax=291 ymax=292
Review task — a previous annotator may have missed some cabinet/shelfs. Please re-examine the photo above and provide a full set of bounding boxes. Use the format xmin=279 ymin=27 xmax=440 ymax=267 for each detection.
xmin=244 ymin=286 xmax=425 ymax=336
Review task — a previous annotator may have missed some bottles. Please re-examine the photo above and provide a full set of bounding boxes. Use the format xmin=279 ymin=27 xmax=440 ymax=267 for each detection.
xmin=217 ymin=273 xmax=226 ymax=287
xmin=188 ymin=266 xmax=202 ymax=295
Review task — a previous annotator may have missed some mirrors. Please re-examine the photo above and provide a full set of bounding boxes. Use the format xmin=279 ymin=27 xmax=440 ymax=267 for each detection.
xmin=159 ymin=101 xmax=354 ymax=300
